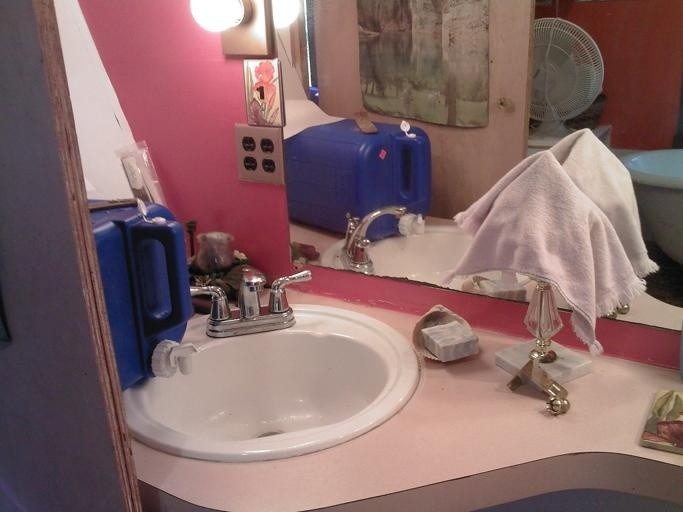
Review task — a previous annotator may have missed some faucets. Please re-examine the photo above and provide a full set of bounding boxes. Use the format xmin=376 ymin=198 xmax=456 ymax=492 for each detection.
xmin=345 ymin=205 xmax=408 ymax=258
xmin=236 ymin=265 xmax=268 ymax=321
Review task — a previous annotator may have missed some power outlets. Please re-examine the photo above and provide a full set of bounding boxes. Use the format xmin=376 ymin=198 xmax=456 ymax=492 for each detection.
xmin=233 ymin=122 xmax=286 ymax=186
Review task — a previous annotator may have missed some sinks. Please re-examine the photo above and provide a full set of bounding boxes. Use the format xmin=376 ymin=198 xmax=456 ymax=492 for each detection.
xmin=122 ymin=301 xmax=422 ymax=465
xmin=369 ymin=229 xmax=534 ymax=292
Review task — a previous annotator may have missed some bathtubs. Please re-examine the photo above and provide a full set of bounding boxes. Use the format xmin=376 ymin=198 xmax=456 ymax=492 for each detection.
xmin=617 ymin=146 xmax=683 ymax=266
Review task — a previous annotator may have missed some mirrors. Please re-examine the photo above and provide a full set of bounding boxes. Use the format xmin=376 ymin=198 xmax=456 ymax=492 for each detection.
xmin=270 ymin=0 xmax=682 ymax=332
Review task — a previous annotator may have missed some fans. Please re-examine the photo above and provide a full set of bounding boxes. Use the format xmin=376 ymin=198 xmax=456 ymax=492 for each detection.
xmin=529 ymin=18 xmax=604 ymax=148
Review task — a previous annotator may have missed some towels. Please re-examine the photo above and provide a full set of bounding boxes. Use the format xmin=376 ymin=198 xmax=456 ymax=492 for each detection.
xmin=547 ymin=126 xmax=660 ymax=278
xmin=442 ymin=149 xmax=647 ymax=359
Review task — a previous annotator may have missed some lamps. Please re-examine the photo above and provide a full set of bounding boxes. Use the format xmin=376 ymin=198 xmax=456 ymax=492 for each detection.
xmin=189 ymin=0 xmax=274 ymax=57
xmin=271 ymin=0 xmax=302 ymax=66
